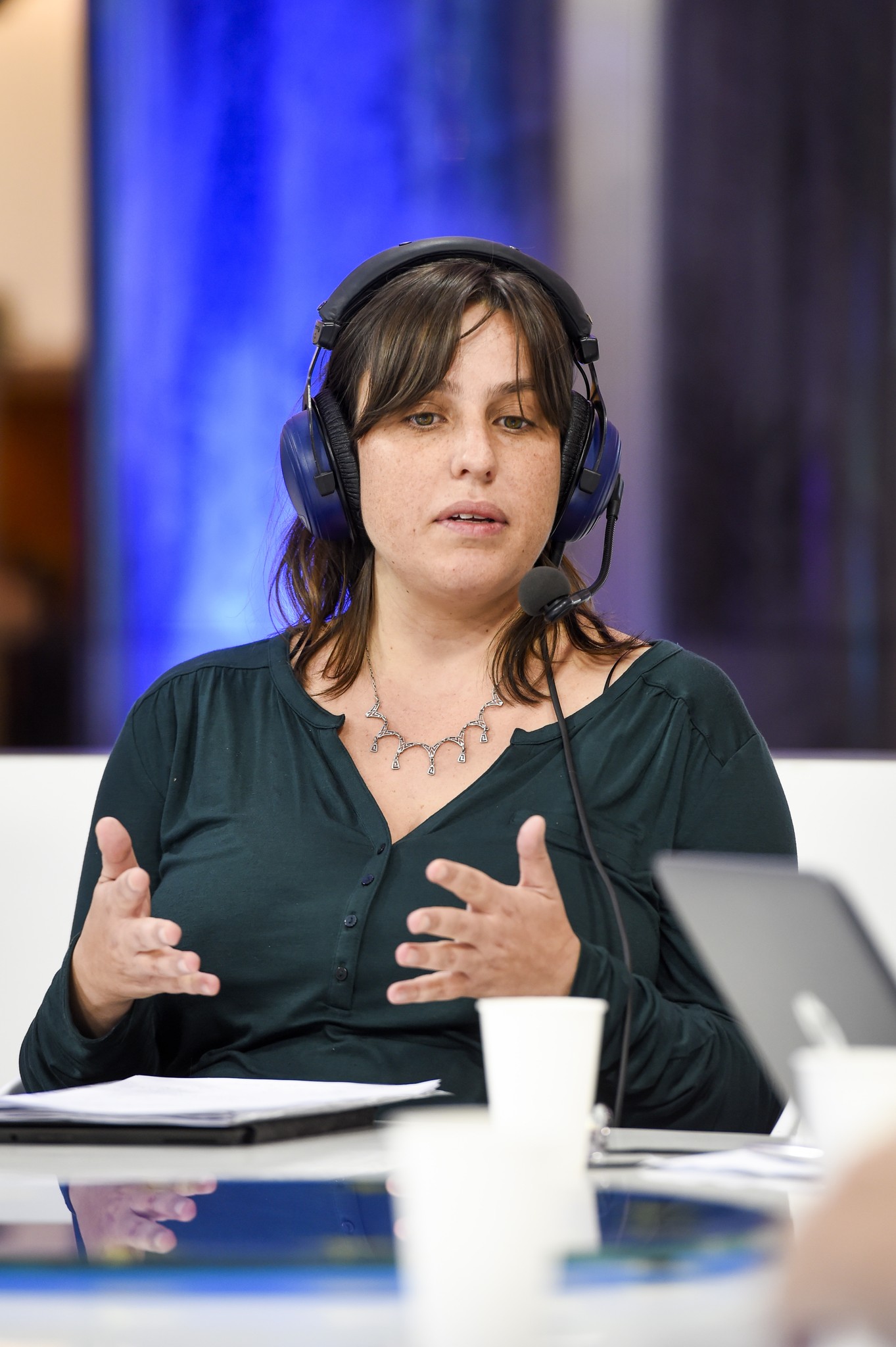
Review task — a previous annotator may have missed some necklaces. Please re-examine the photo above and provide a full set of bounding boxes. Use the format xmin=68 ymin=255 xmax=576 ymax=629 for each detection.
xmin=362 ymin=645 xmax=510 ymax=772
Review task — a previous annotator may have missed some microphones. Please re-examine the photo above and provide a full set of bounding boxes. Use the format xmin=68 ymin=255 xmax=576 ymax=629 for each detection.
xmin=516 ymin=477 xmax=625 ymax=626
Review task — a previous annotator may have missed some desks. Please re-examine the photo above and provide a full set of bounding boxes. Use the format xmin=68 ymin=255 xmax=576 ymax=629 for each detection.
xmin=0 ymin=1105 xmax=824 ymax=1347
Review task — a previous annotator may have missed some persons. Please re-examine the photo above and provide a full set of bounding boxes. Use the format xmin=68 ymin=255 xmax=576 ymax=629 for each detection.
xmin=1 ymin=1172 xmax=221 ymax=1267
xmin=12 ymin=243 xmax=809 ymax=1139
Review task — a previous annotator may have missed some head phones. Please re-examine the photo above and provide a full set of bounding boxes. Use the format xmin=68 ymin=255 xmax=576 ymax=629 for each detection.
xmin=278 ymin=237 xmax=624 ymax=553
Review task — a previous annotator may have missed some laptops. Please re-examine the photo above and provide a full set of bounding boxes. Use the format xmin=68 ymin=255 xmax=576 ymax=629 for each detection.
xmin=643 ymin=852 xmax=896 ymax=1109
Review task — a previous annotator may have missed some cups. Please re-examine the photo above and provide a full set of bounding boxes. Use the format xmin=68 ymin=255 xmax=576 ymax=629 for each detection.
xmin=471 ymin=994 xmax=607 ymax=1150
xmin=387 ymin=1110 xmax=563 ymax=1330
